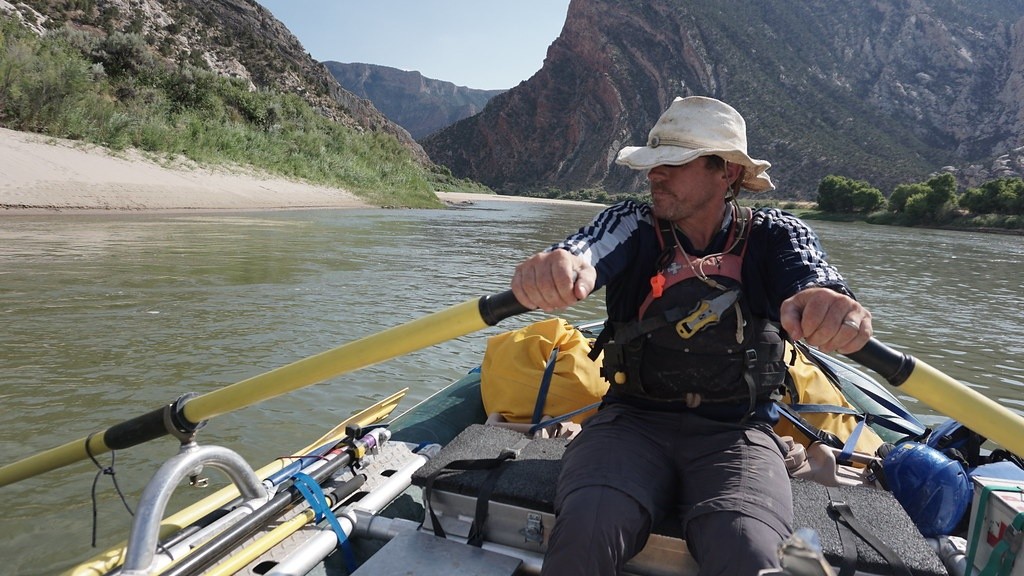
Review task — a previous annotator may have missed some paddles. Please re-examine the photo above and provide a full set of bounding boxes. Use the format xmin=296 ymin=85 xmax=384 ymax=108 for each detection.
xmin=1 ymin=285 xmax=528 ymax=487
xmin=849 ymin=326 xmax=1023 ymax=466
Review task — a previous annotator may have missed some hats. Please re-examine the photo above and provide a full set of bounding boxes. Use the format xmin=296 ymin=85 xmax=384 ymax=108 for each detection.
xmin=613 ymin=94 xmax=775 ymax=193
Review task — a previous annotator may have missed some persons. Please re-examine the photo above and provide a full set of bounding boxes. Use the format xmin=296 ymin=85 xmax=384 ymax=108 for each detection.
xmin=510 ymin=95 xmax=873 ymax=576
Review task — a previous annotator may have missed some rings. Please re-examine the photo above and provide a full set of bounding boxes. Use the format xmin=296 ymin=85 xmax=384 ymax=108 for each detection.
xmin=842 ymin=320 xmax=859 ymax=331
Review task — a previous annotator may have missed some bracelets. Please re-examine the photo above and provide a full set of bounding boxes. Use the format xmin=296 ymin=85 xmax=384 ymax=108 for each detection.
xmin=838 ymin=286 xmax=854 ymax=298
